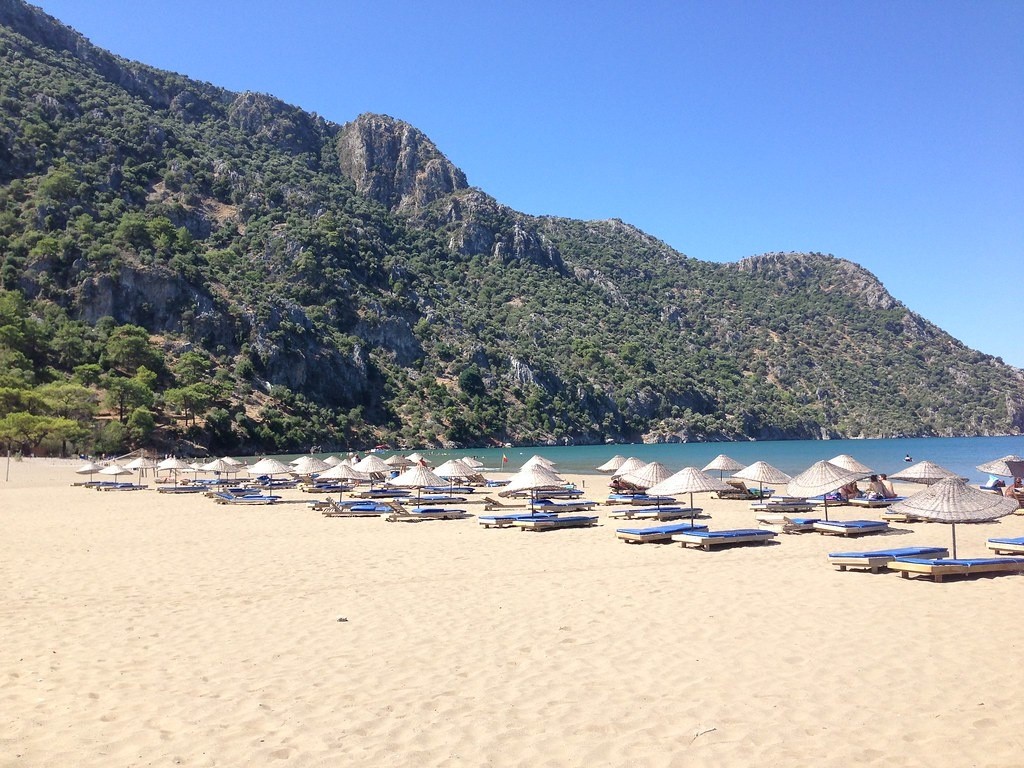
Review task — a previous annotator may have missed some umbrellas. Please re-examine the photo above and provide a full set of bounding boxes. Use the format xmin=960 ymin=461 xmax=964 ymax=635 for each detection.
xmin=786 ymin=459 xmax=872 ymax=522
xmin=828 ymin=454 xmax=875 ymax=474
xmin=887 ymin=460 xmax=969 ymax=488
xmin=643 ymin=464 xmax=741 ymax=530
xmin=499 ymin=454 xmax=573 ymax=517
xmin=702 ymin=453 xmax=747 ymax=499
xmin=77 ymin=451 xmax=341 ymax=497
xmin=595 ymin=453 xmax=675 ymax=509
xmin=975 ymin=455 xmax=1024 ymax=477
xmin=385 ymin=455 xmax=483 ymax=509
xmin=312 ymin=451 xmax=432 ymax=502
xmin=732 ymin=460 xmax=793 ymax=509
xmin=886 ymin=477 xmax=1019 ymax=560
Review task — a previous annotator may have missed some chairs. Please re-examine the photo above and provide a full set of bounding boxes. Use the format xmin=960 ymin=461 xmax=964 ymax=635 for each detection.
xmin=70 ymin=474 xmax=1024 ymax=583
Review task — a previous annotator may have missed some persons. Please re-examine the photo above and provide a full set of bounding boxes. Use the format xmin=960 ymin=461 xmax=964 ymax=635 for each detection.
xmin=1004 ymin=477 xmax=1022 ymax=498
xmin=355 ymin=453 xmax=361 ymax=462
xmin=417 ymin=457 xmax=427 ymax=468
xmin=401 ymin=453 xmax=405 ymax=458
xmin=347 ymin=448 xmax=353 ymax=458
xmin=840 ymin=473 xmax=897 ymax=498
xmin=429 ymin=466 xmax=435 ymax=471
xmin=613 ymin=480 xmax=644 ymax=490
xmin=905 ymin=454 xmax=912 ymax=462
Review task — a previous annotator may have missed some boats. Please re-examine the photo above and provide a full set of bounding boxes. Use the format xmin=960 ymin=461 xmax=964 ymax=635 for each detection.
xmin=505 ymin=442 xmax=512 ymax=449
xmin=370 ymin=448 xmax=386 ymax=455
